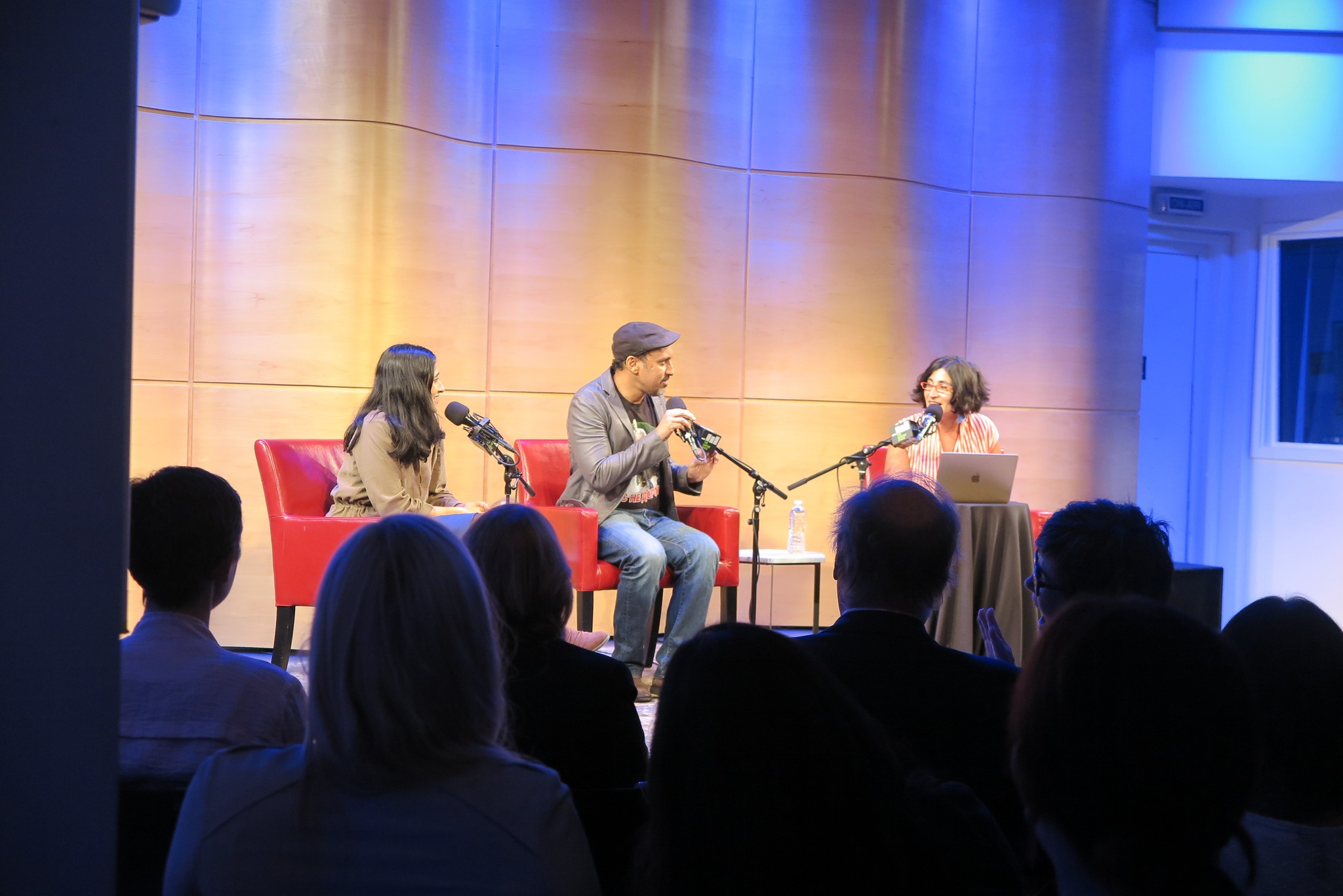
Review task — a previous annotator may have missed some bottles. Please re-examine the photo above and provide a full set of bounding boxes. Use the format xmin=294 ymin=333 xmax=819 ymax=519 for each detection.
xmin=787 ymin=499 xmax=806 ymax=555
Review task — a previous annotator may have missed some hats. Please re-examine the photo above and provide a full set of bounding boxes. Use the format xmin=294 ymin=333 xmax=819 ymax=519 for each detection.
xmin=612 ymin=321 xmax=680 ymax=359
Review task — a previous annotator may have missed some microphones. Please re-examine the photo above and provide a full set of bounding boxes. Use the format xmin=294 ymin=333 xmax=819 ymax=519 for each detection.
xmin=666 ymin=396 xmax=701 ymax=459
xmin=444 ymin=401 xmax=518 ymax=456
xmin=915 ymin=404 xmax=943 ymax=443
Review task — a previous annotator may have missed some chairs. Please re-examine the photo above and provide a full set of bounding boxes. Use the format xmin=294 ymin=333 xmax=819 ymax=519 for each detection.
xmin=253 ymin=438 xmax=383 ymax=670
xmin=515 ymin=439 xmax=740 ymax=666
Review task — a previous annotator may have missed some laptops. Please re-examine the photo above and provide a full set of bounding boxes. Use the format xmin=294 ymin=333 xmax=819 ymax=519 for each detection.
xmin=933 ymin=452 xmax=1018 ymax=503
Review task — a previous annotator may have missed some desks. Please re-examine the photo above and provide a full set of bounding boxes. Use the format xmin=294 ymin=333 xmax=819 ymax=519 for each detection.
xmin=923 ymin=502 xmax=1042 ymax=666
xmin=735 ymin=548 xmax=826 ymax=635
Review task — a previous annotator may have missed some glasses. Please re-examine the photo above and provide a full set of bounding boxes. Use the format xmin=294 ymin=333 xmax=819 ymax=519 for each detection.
xmin=920 ymin=382 xmax=954 ymax=393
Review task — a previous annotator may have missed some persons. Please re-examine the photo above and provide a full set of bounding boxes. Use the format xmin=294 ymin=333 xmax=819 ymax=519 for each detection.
xmin=324 ymin=343 xmax=490 ymax=519
xmin=788 ymin=470 xmax=1060 ymax=896
xmin=1005 ymin=594 xmax=1259 ymax=896
xmin=1220 ymin=596 xmax=1343 ymax=896
xmin=461 ymin=502 xmax=650 ymax=855
xmin=553 ymin=321 xmax=720 ymax=701
xmin=163 ymin=514 xmax=599 ymax=896
xmin=978 ymin=494 xmax=1174 ymax=672
xmin=118 ymin=464 xmax=305 ymax=744
xmin=617 ymin=620 xmax=944 ymax=896
xmin=884 ymin=356 xmax=1003 ymax=495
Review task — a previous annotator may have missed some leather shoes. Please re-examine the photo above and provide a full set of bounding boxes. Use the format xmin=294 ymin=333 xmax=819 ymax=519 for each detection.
xmin=632 ymin=677 xmax=652 ymax=702
xmin=650 ymin=678 xmax=664 ymax=696
xmin=564 ymin=627 xmax=610 ymax=651
